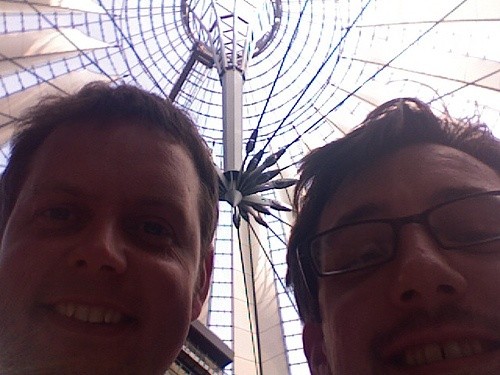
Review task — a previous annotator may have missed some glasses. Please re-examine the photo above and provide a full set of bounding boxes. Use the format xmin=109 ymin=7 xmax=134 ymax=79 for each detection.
xmin=295 ymin=190 xmax=500 ymax=325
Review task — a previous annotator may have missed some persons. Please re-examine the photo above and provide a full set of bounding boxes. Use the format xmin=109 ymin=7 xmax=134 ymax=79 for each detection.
xmin=283 ymin=93 xmax=499 ymax=375
xmin=0 ymin=79 xmax=220 ymax=375
